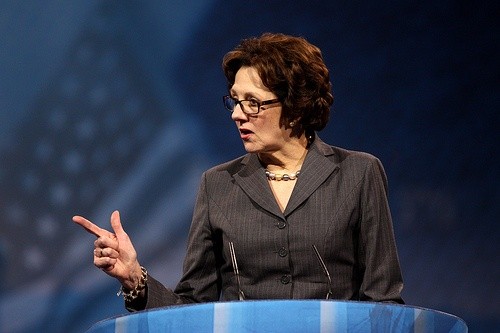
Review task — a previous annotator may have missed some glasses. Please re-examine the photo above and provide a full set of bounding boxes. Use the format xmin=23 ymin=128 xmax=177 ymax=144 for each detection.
xmin=222 ymin=93 xmax=281 ymax=115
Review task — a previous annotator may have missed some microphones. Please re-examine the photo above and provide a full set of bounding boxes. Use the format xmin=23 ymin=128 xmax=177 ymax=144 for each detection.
xmin=313 ymin=244 xmax=335 ymax=300
xmin=228 ymin=241 xmax=245 ymax=301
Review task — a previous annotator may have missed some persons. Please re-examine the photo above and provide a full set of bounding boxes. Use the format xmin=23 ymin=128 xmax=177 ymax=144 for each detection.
xmin=72 ymin=31 xmax=408 ymax=307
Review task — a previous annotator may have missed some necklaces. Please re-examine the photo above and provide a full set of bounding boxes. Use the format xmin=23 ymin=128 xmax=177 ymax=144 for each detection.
xmin=264 ymin=169 xmax=302 ymax=182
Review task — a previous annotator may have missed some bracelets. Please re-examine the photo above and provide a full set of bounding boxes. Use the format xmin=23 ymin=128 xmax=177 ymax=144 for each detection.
xmin=117 ymin=266 xmax=148 ymax=303
